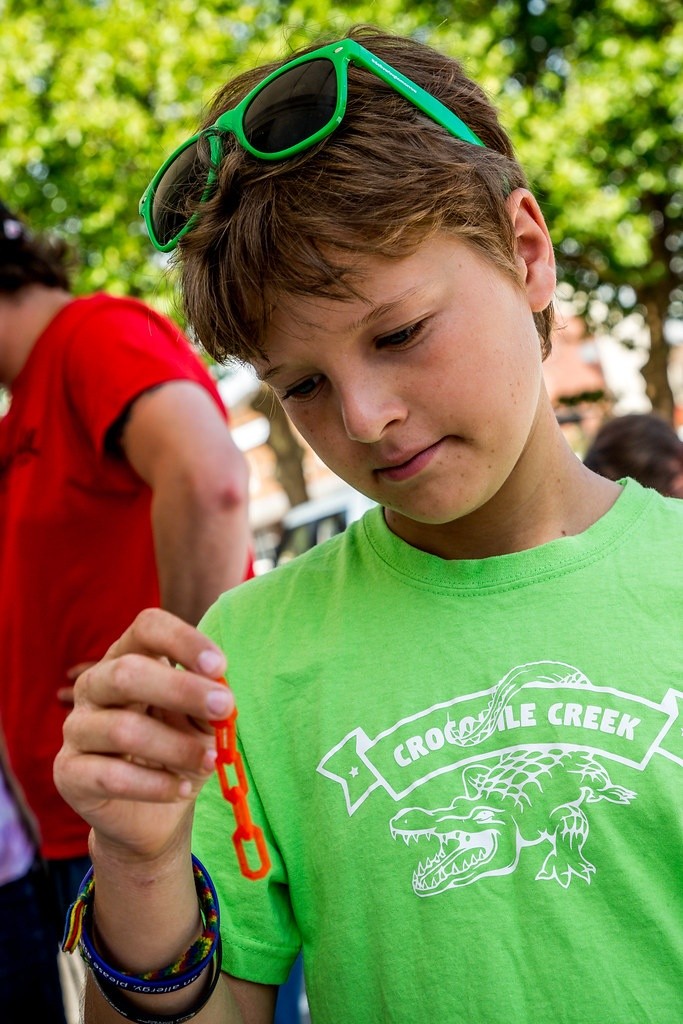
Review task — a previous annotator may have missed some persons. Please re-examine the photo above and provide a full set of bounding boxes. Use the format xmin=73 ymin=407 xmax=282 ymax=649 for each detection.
xmin=1 ymin=200 xmax=253 ymax=1024
xmin=50 ymin=24 xmax=682 ymax=1024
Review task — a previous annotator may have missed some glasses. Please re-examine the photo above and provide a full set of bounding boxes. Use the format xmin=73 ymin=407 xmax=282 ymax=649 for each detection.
xmin=138 ymin=39 xmax=511 ymax=253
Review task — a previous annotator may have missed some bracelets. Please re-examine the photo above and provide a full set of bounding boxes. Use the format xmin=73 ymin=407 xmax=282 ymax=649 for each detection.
xmin=87 ymin=933 xmax=223 ymax=1024
xmin=60 ymin=852 xmax=220 ymax=994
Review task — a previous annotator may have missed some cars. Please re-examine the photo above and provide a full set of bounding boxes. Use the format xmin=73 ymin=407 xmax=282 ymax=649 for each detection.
xmin=270 ymin=483 xmax=374 ymax=573
